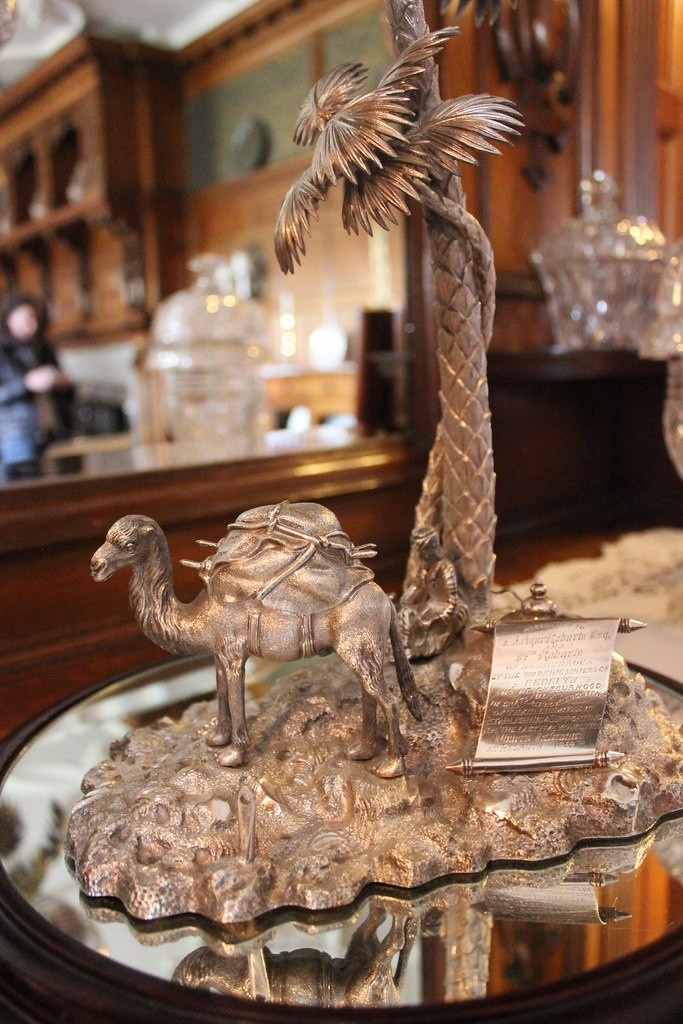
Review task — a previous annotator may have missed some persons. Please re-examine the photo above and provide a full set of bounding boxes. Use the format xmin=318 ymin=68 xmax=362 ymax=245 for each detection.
xmin=0 ymin=296 xmax=85 ymax=483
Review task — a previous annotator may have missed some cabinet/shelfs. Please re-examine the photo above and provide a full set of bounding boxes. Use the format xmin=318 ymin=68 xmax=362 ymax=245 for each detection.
xmin=1 ymin=32 xmax=177 ymax=368
